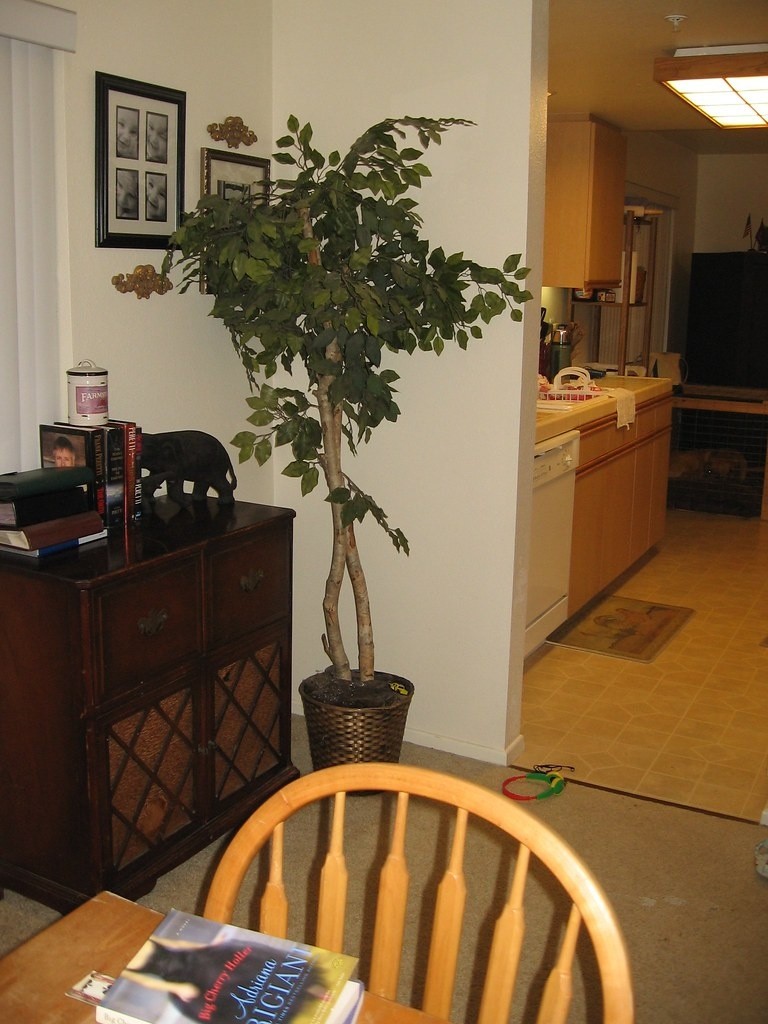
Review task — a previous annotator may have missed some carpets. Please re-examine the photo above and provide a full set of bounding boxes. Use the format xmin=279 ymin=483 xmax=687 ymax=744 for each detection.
xmin=546 ymin=593 xmax=697 ymax=662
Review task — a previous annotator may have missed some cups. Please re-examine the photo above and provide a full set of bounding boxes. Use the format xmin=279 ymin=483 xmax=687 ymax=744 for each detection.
xmin=66 ymin=358 xmax=109 ymax=426
xmin=549 ymin=325 xmax=571 ymax=383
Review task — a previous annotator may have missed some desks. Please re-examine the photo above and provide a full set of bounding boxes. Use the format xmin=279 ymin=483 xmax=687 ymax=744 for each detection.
xmin=0 ymin=890 xmax=457 ymax=1024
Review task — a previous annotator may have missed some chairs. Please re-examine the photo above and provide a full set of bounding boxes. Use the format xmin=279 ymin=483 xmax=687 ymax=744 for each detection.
xmin=202 ymin=763 xmax=635 ymax=1024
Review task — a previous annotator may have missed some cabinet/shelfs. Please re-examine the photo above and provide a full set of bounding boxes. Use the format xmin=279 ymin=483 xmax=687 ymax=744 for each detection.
xmin=542 ymin=111 xmax=626 ymax=289
xmin=565 ymin=210 xmax=659 ymax=378
xmin=0 ymin=494 xmax=300 ymax=918
xmin=567 ymin=395 xmax=675 ymax=624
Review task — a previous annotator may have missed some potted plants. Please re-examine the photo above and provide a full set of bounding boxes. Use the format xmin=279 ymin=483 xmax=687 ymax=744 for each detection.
xmin=156 ymin=115 xmax=533 ymax=794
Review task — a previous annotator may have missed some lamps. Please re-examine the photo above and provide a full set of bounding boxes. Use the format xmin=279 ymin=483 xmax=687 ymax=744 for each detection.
xmin=654 ymin=43 xmax=768 ymax=131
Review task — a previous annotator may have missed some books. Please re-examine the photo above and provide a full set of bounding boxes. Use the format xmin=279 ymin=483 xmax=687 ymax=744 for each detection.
xmin=0 ymin=419 xmax=142 ymax=559
xmin=94 ymin=907 xmax=365 ymax=1024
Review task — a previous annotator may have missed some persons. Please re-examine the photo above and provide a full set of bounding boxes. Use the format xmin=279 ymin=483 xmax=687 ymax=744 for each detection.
xmin=52 ymin=436 xmax=76 ymax=467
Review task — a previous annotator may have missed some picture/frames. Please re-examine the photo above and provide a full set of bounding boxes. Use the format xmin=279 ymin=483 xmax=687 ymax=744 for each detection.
xmin=199 ymin=147 xmax=270 ymax=295
xmin=95 ymin=71 xmax=186 ymax=250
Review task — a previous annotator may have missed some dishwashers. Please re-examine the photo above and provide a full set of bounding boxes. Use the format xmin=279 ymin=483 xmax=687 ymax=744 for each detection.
xmin=524 ymin=429 xmax=581 ymax=659
